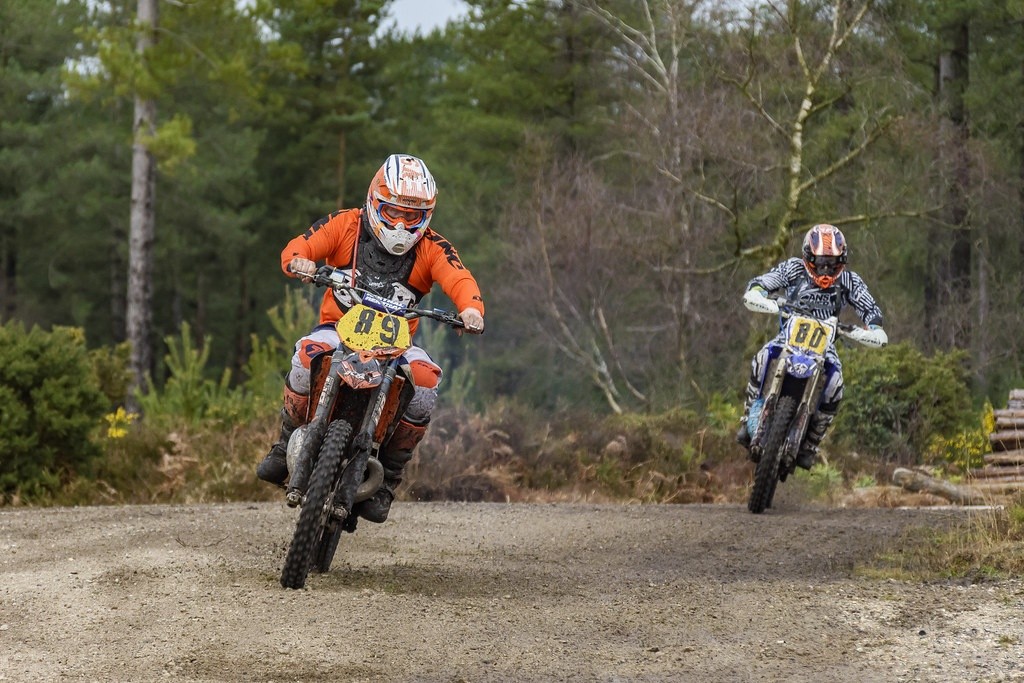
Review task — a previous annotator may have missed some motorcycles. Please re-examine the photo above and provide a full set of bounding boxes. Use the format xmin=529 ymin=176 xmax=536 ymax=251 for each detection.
xmin=748 ymin=296 xmax=857 ymax=513
xmin=278 ymin=262 xmax=485 ymax=590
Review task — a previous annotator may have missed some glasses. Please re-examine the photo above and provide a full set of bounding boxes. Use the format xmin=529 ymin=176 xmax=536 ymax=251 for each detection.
xmin=810 ymin=255 xmax=843 ymax=275
xmin=378 ymin=202 xmax=426 ymax=229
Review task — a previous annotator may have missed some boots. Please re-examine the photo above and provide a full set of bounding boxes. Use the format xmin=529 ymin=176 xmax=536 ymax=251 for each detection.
xmin=738 ymin=379 xmax=760 ymax=441
xmin=359 ymin=416 xmax=430 ymax=523
xmin=257 ymin=371 xmax=309 ymax=483
xmin=797 ymin=409 xmax=836 ymax=470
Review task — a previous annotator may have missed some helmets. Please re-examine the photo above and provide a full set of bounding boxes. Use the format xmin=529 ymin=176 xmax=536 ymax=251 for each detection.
xmin=802 ymin=224 xmax=847 ymax=288
xmin=366 ymin=154 xmax=439 ymax=255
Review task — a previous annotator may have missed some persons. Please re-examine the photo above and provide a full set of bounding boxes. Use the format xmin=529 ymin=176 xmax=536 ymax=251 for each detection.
xmin=256 ymin=153 xmax=485 ymax=524
xmin=736 ymin=223 xmax=888 ymax=470
